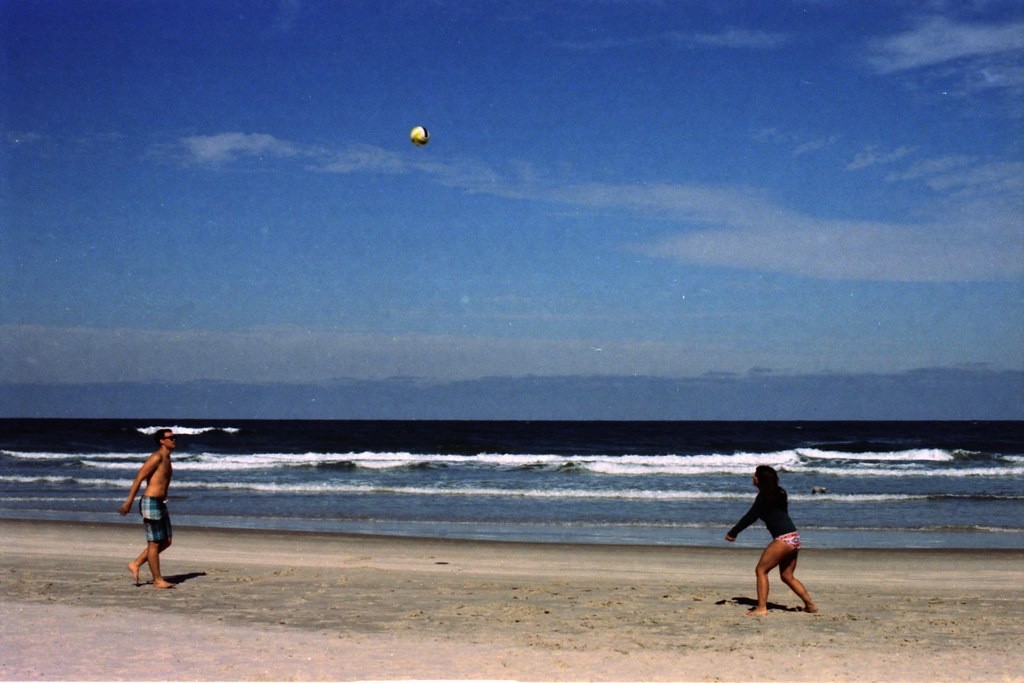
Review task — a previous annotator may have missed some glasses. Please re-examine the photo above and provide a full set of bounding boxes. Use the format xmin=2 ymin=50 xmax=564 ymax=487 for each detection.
xmin=164 ymin=434 xmax=177 ymax=441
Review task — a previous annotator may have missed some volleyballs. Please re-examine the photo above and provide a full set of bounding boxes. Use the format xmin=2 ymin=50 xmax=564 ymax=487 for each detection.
xmin=410 ymin=126 xmax=430 ymax=147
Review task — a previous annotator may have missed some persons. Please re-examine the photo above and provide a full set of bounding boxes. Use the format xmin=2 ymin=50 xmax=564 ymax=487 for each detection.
xmin=121 ymin=429 xmax=176 ymax=588
xmin=725 ymin=465 xmax=817 ymax=615
